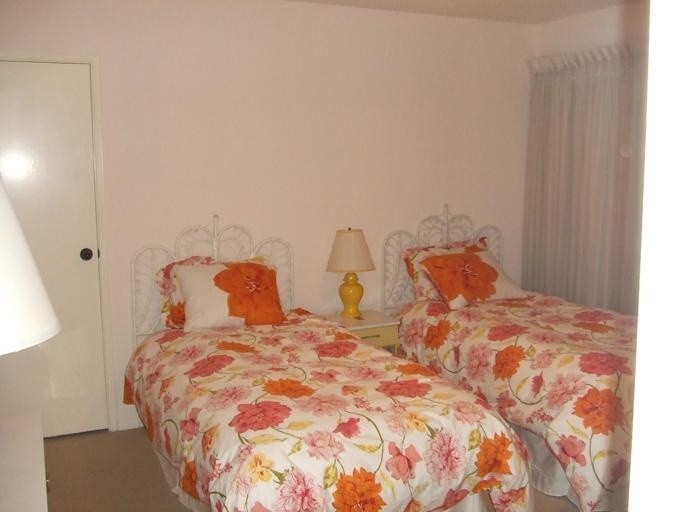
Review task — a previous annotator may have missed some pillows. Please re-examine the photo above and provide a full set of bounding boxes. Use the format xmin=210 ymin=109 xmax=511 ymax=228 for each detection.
xmin=403 ymin=236 xmax=488 ymax=299
xmin=154 ymin=256 xmax=267 ymax=328
xmin=420 ymin=251 xmax=529 ymax=309
xmin=169 ymin=264 xmax=284 ymax=333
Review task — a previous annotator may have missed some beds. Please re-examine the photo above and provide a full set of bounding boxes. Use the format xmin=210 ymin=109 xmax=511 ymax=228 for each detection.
xmin=383 ymin=204 xmax=638 ymax=512
xmin=123 ymin=214 xmax=531 ymax=512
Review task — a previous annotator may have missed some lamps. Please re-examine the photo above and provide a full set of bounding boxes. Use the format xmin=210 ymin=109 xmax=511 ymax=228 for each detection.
xmin=327 ymin=228 xmax=375 ymax=318
xmin=1 ymin=173 xmax=61 ymax=361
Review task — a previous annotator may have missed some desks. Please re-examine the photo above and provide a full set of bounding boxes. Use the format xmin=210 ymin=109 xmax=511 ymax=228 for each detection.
xmin=1 ymin=410 xmax=49 ymax=512
xmin=329 ymin=310 xmax=400 ymax=356
